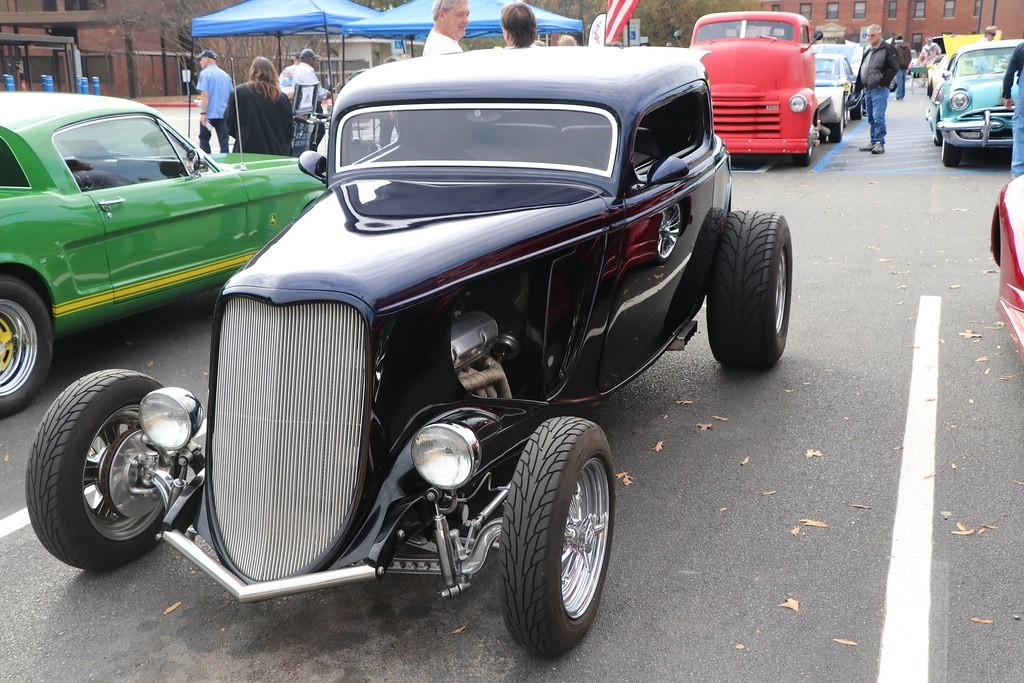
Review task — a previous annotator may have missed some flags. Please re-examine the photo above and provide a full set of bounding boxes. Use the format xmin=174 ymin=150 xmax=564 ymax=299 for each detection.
xmin=605 ymin=0 xmax=640 ymax=45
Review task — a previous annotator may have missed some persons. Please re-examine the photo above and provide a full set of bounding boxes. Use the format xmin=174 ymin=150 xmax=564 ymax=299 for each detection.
xmin=972 ymin=25 xmax=996 ymax=72
xmin=893 ymin=33 xmax=912 ymax=99
xmin=293 ymin=48 xmax=331 ymax=153
xmin=1003 ymin=41 xmax=1024 ymax=177
xmin=196 ymin=50 xmax=233 ymax=153
xmin=279 ymin=51 xmax=301 ymax=96
xmin=379 ymin=0 xmax=578 ymax=149
xmin=916 ymin=36 xmax=942 ymax=87
xmin=855 ymin=24 xmax=899 ymax=154
xmin=224 ymin=56 xmax=294 ymax=157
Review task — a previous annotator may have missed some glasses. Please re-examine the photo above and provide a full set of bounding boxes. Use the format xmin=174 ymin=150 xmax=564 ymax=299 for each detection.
xmin=991 ymin=34 xmax=996 ymax=36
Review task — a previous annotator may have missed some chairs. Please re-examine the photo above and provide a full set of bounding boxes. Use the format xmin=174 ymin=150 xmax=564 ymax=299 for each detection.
xmin=559 ymin=122 xmax=656 ymax=173
xmin=487 ymin=121 xmax=562 ymax=165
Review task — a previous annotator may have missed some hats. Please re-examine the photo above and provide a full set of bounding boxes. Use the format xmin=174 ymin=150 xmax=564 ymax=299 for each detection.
xmin=894 ymin=34 xmax=905 ymax=43
xmin=198 ymin=50 xmax=219 ymax=62
xmin=299 ymin=49 xmax=320 ymax=61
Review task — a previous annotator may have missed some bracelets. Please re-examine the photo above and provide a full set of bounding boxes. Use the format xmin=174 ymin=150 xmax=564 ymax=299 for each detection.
xmin=201 ymin=112 xmax=206 ymax=115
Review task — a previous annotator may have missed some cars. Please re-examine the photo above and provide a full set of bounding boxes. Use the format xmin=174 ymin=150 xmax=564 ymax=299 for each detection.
xmin=908 ymin=49 xmax=919 ymax=67
xmin=0 ymin=90 xmax=325 ymax=419
xmin=926 ymin=39 xmax=1024 ymax=169
xmin=25 ymin=45 xmax=793 ymax=656
xmin=813 ymin=53 xmax=866 ymax=120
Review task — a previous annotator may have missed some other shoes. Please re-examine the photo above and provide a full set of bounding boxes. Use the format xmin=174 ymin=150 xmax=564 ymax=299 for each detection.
xmin=871 ymin=143 xmax=884 ymax=154
xmin=859 ymin=143 xmax=876 ymax=151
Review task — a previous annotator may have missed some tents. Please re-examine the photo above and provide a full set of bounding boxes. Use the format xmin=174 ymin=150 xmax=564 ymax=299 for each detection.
xmin=340 ymin=0 xmax=583 ymax=86
xmin=187 ymin=0 xmax=404 ymax=138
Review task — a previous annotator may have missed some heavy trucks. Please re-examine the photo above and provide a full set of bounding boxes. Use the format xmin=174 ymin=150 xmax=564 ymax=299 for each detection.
xmin=672 ymin=10 xmax=846 ymax=166
